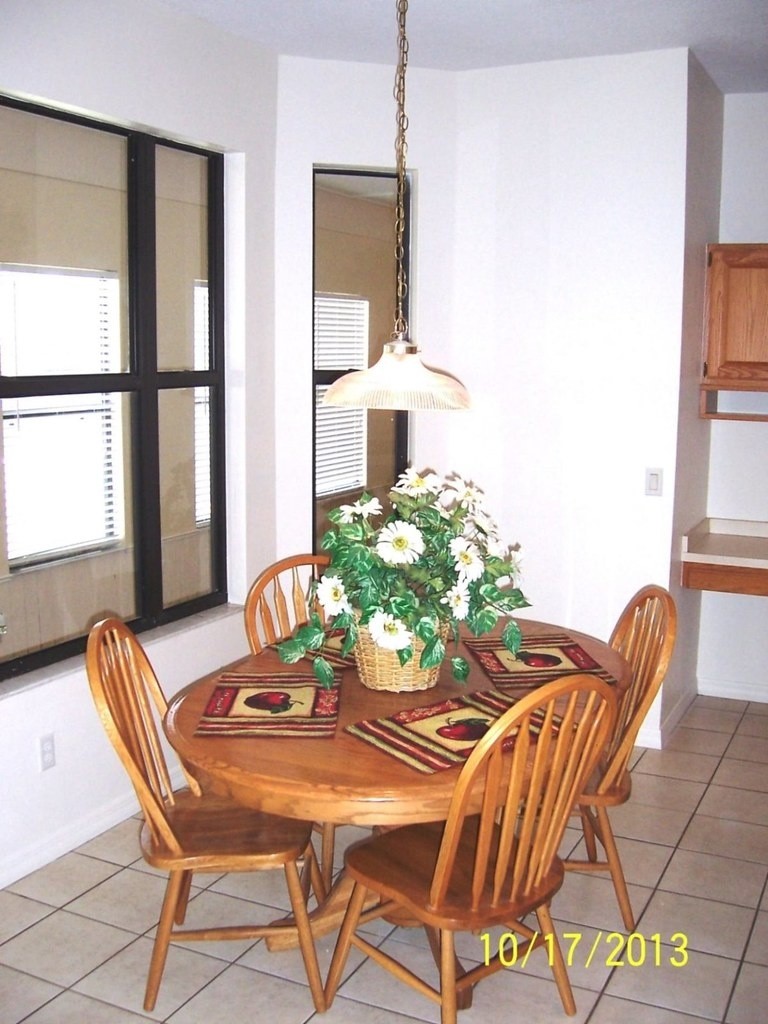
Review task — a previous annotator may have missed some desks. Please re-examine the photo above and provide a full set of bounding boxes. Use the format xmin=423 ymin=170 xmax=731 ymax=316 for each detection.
xmin=164 ymin=616 xmax=636 ymax=1011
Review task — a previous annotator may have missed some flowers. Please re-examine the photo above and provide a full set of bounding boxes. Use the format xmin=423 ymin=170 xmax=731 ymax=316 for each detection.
xmin=274 ymin=467 xmax=529 ymax=685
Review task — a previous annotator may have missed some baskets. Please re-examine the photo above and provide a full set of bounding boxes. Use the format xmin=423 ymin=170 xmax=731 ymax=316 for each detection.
xmin=348 ymin=605 xmax=451 ymax=692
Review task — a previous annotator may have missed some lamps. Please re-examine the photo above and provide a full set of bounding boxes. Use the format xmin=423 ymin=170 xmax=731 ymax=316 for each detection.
xmin=322 ymin=0 xmax=471 ymax=412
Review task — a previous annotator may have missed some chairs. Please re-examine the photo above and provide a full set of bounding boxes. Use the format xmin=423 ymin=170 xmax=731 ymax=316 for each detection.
xmin=85 ymin=617 xmax=329 ymax=1014
xmin=504 ymin=582 xmax=677 ymax=932
xmin=245 ymin=555 xmax=339 ymax=901
xmin=323 ymin=674 xmax=618 ymax=1023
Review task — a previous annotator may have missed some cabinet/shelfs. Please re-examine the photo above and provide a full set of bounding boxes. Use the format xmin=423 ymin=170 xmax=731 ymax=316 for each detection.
xmin=702 ymin=243 xmax=768 ymax=389
